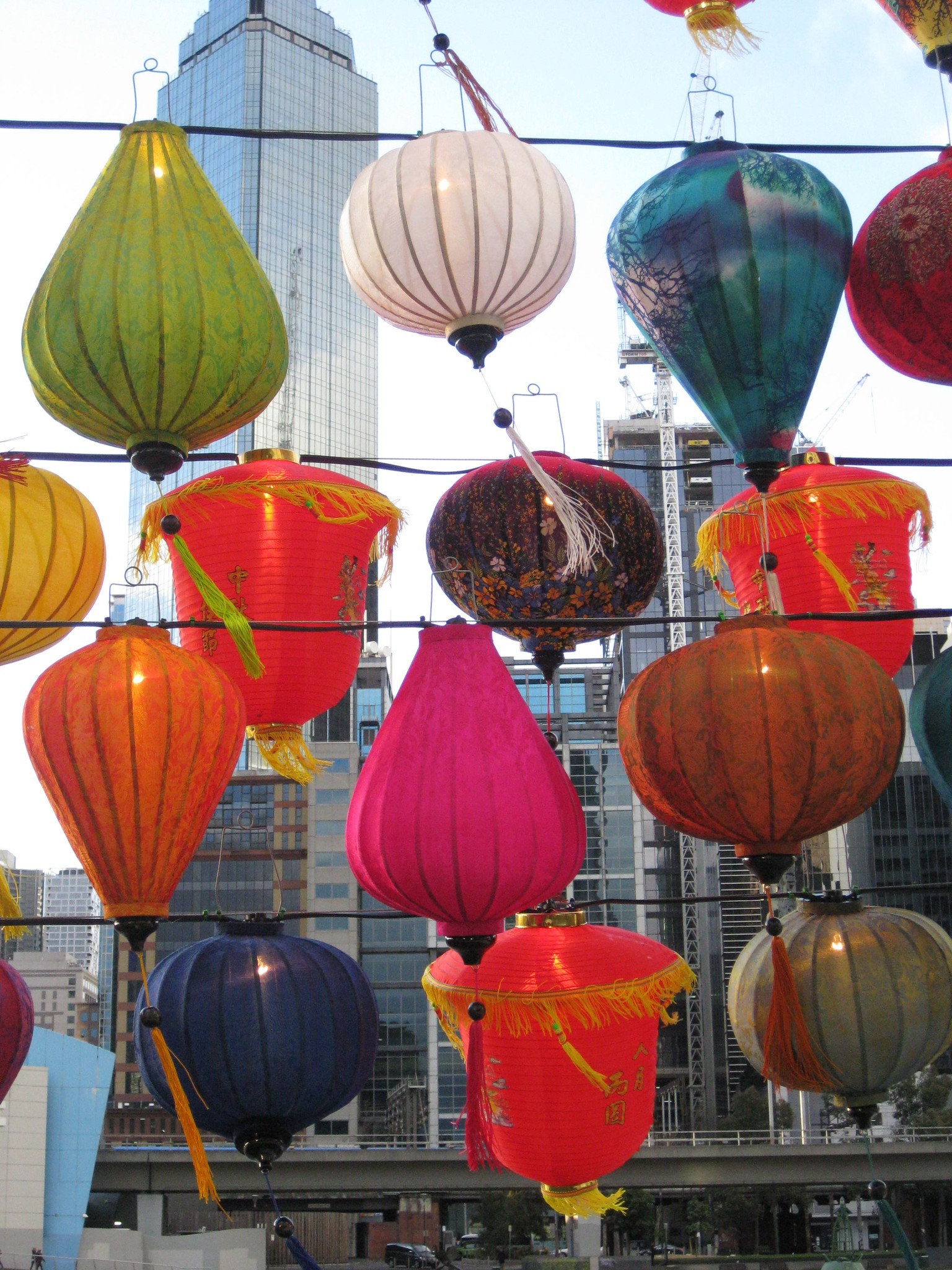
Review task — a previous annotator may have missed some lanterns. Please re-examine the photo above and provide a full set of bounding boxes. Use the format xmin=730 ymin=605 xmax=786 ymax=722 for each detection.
xmin=642 ymin=0 xmax=763 ymax=59
xmin=876 ymin=0 xmax=951 ymax=83
xmin=0 ymin=61 xmax=950 ymax=1221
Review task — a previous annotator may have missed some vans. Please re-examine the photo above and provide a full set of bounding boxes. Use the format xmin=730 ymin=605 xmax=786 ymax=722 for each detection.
xmin=384 ymin=1243 xmax=440 ymax=1270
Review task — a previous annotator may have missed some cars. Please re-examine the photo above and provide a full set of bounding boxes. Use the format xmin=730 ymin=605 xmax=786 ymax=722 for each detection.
xmin=548 ymin=1247 xmax=568 ymax=1258
xmin=636 ymin=1244 xmax=683 ymax=1255
xmin=446 ymin=1233 xmax=482 ymax=1250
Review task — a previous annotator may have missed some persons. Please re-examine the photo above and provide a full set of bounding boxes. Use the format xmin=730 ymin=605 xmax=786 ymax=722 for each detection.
xmin=496 ymin=1247 xmax=506 ymax=1270
